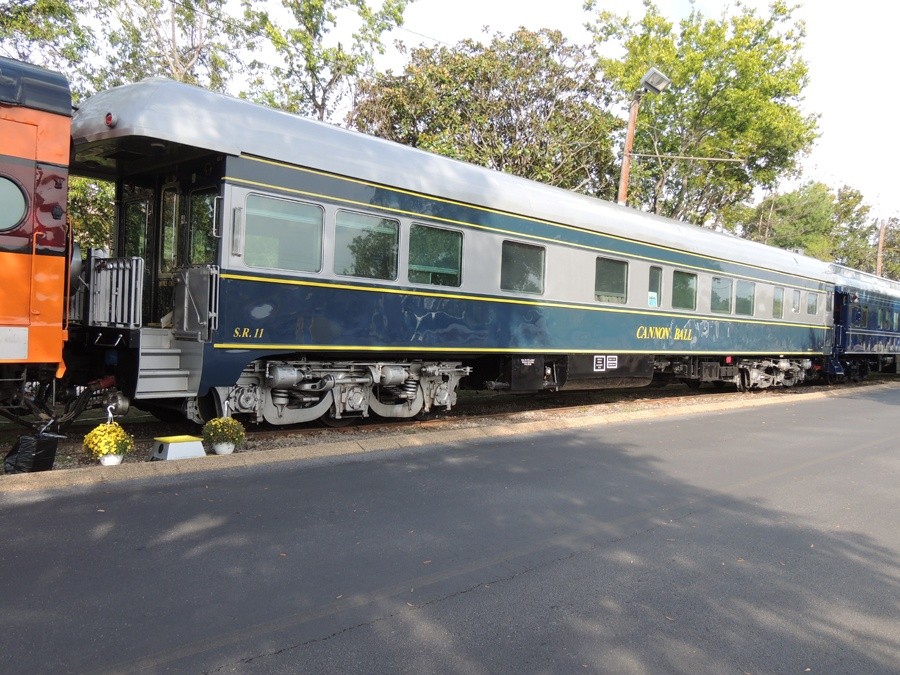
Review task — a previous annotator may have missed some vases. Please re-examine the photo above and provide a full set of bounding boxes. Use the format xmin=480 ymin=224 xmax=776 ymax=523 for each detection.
xmin=212 ymin=442 xmax=237 ymax=455
xmin=98 ymin=454 xmax=125 ymax=467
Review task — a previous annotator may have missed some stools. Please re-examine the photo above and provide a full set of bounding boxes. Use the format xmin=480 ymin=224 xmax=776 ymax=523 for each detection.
xmin=147 ymin=434 xmax=208 ymax=462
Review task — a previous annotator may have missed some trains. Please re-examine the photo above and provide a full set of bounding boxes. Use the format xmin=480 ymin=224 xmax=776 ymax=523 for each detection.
xmin=0 ymin=51 xmax=900 ymax=436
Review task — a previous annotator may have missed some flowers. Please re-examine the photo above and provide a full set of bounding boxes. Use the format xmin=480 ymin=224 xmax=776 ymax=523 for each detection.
xmin=81 ymin=421 xmax=136 ymax=462
xmin=201 ymin=415 xmax=249 ymax=447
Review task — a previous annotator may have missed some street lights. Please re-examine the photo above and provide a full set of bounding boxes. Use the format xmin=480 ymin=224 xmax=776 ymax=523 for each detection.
xmin=616 ymin=66 xmax=671 ymax=207
